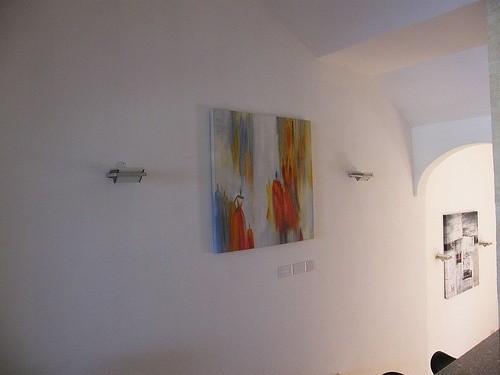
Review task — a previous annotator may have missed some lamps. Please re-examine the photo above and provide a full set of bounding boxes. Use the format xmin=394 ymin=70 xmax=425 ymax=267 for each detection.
xmin=478 ymin=240 xmax=493 ymax=248
xmin=105 ymin=160 xmax=148 ymax=184
xmin=435 ymin=254 xmax=452 ymax=262
xmin=347 ymin=166 xmax=374 ymax=182
